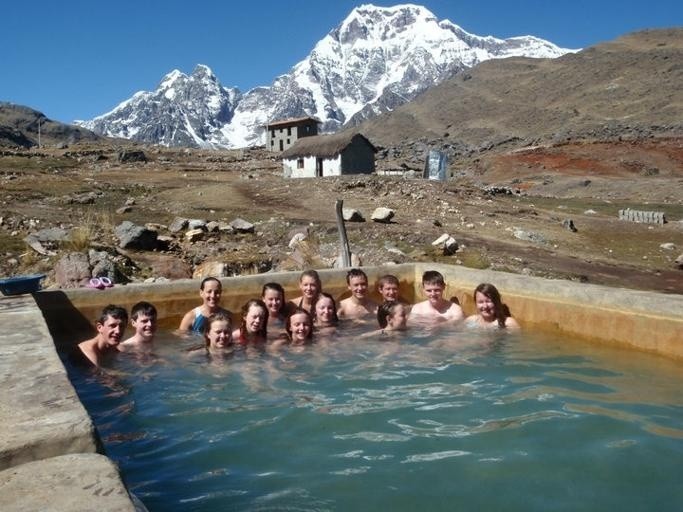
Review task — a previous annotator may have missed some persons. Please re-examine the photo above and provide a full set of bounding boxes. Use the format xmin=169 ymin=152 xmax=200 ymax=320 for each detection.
xmin=178 ymin=266 xmax=519 ymax=358
xmin=122 ymin=302 xmax=166 ymax=351
xmin=71 ymin=304 xmax=157 ymax=379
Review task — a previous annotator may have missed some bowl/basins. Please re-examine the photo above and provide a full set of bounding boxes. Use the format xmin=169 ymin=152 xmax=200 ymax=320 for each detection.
xmin=0 ymin=274 xmax=48 ymax=296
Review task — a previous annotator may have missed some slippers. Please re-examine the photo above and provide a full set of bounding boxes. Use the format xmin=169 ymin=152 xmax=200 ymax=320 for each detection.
xmin=100 ymin=277 xmax=111 ymax=287
xmin=89 ymin=278 xmax=101 ymax=288
